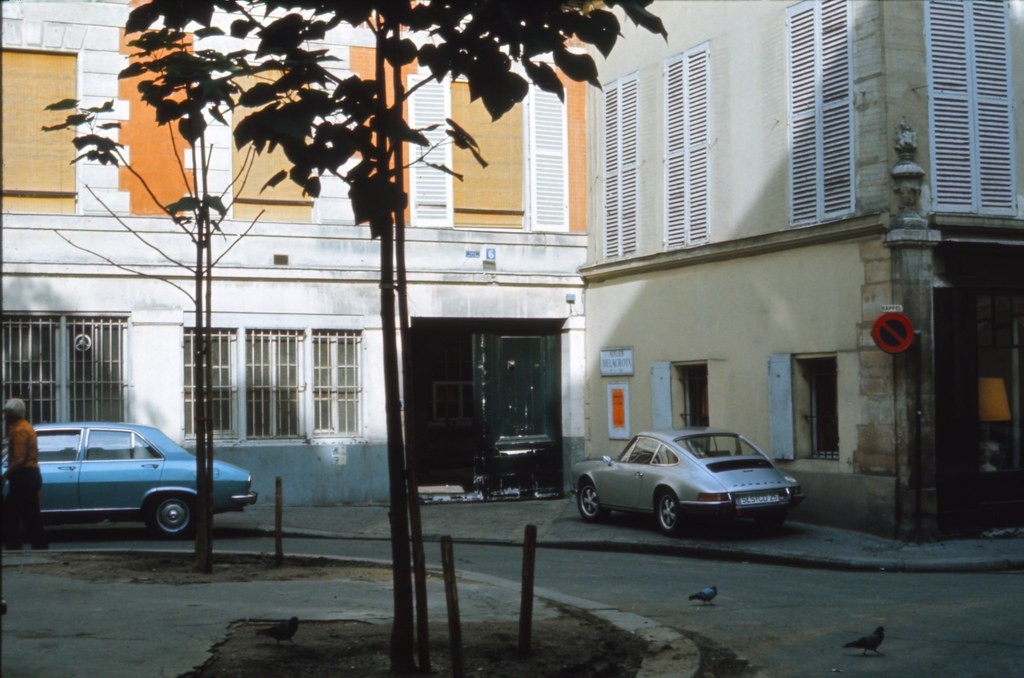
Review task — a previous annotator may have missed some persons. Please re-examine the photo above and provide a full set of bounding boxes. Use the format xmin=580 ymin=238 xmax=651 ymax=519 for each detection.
xmin=0 ymin=398 xmax=48 ymax=550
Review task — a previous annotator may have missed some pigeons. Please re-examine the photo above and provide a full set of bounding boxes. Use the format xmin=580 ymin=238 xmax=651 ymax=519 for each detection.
xmin=255 ymin=616 xmax=299 ymax=645
xmin=843 ymin=626 xmax=886 ymax=656
xmin=688 ymin=586 xmax=718 ymax=606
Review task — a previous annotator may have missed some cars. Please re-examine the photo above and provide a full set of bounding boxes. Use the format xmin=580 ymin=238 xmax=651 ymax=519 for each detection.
xmin=569 ymin=425 xmax=807 ymax=538
xmin=2 ymin=421 xmax=260 ymax=540
